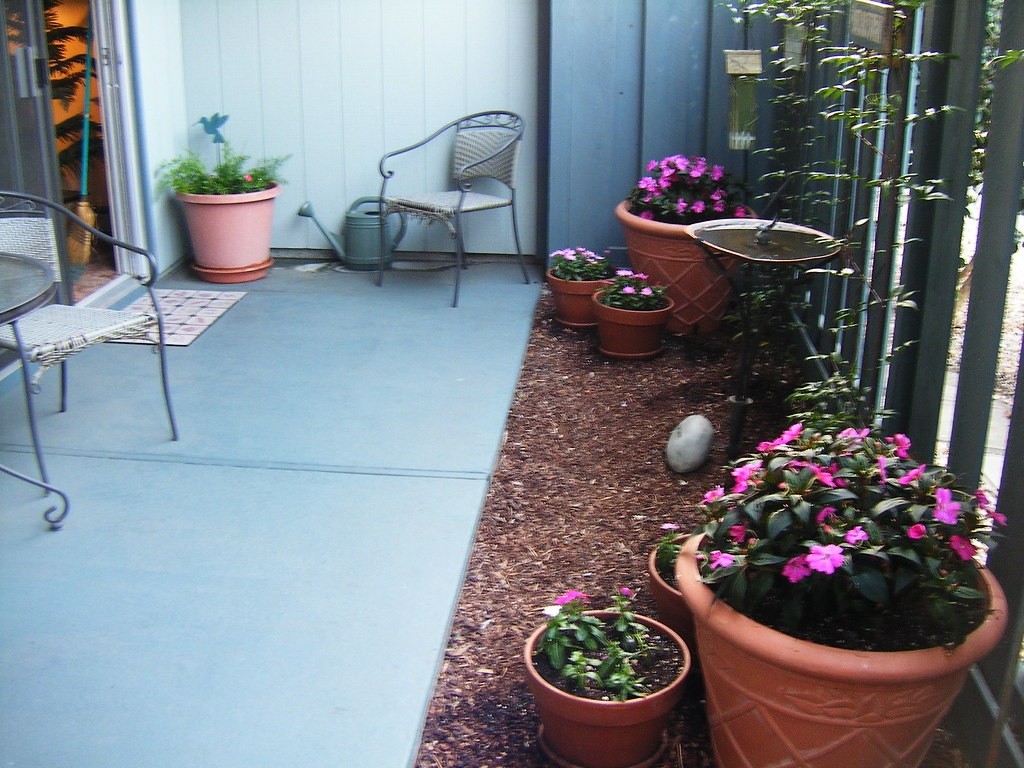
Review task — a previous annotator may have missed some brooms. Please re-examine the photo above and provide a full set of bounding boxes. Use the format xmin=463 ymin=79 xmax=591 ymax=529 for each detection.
xmin=66 ymin=4 xmax=96 ymax=265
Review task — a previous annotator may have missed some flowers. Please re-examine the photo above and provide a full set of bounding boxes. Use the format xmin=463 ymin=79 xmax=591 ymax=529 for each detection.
xmin=625 ymin=154 xmax=752 ymax=224
xmin=539 ymin=583 xmax=658 ymax=700
xmin=548 ymin=244 xmax=611 ymax=282
xmin=152 ymin=140 xmax=293 ymax=192
xmin=595 ymin=270 xmax=670 ymax=311
xmin=658 ymin=522 xmax=682 ymax=567
xmin=688 ymin=423 xmax=1012 ymax=649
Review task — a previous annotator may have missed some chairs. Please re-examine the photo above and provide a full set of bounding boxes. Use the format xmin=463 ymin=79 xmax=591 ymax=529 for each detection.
xmin=0 ymin=191 xmax=178 ymax=442
xmin=375 ymin=112 xmax=530 ymax=307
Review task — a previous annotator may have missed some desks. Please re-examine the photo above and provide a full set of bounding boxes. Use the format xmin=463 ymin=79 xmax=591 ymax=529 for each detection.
xmin=0 ymin=251 xmax=70 ymax=533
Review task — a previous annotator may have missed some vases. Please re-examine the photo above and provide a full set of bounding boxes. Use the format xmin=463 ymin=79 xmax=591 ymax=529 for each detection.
xmin=592 ymin=291 xmax=674 ymax=360
xmin=524 ymin=610 xmax=691 ymax=768
xmin=546 ymin=268 xmax=620 ymax=326
xmin=621 ymin=204 xmax=758 ymax=336
xmin=676 ymin=518 xmax=1008 ymax=768
xmin=177 ymin=180 xmax=282 ymax=282
xmin=647 ymin=533 xmax=700 ymax=665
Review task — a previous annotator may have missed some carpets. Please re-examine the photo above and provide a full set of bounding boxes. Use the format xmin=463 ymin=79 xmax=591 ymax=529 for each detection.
xmin=106 ymin=290 xmax=247 ymax=348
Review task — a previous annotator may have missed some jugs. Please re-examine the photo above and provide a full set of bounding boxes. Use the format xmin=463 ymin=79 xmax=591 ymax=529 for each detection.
xmin=299 ymin=197 xmax=408 ymax=270
